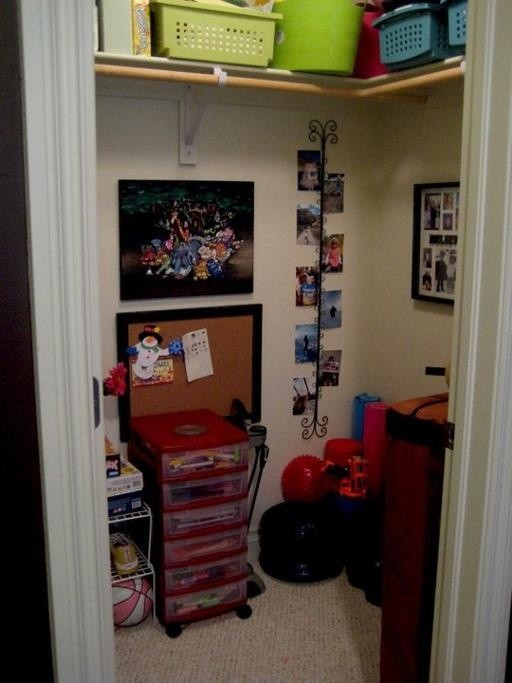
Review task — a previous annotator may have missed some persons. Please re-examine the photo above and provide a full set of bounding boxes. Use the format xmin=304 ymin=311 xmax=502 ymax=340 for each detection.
xmin=422 ymin=193 xmax=448 ymax=293
xmin=296 ymin=269 xmax=317 ymax=305
xmin=321 ymin=354 xmax=335 ymax=385
xmin=325 ymin=237 xmax=343 ymax=271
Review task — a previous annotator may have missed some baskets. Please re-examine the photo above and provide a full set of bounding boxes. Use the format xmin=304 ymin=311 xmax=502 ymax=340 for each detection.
xmin=370 ymin=1 xmax=464 ymax=72
xmin=149 ymin=1 xmax=284 ymax=68
xmin=438 ymin=0 xmax=467 ymax=46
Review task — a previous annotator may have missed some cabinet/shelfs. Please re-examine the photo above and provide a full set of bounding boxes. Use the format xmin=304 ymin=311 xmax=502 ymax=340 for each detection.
xmin=109 ymin=500 xmax=161 ymax=630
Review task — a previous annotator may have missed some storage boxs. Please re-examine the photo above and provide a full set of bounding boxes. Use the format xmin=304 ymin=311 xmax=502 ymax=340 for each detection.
xmin=126 ymin=410 xmax=252 ymax=639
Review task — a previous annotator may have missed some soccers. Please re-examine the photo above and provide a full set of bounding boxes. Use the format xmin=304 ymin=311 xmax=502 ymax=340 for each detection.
xmin=111 ymin=577 xmax=153 ymax=626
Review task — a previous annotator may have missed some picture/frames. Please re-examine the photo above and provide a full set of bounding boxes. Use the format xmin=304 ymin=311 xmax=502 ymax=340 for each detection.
xmin=410 ymin=180 xmax=461 ymax=304
xmin=115 ymin=302 xmax=263 ymax=443
